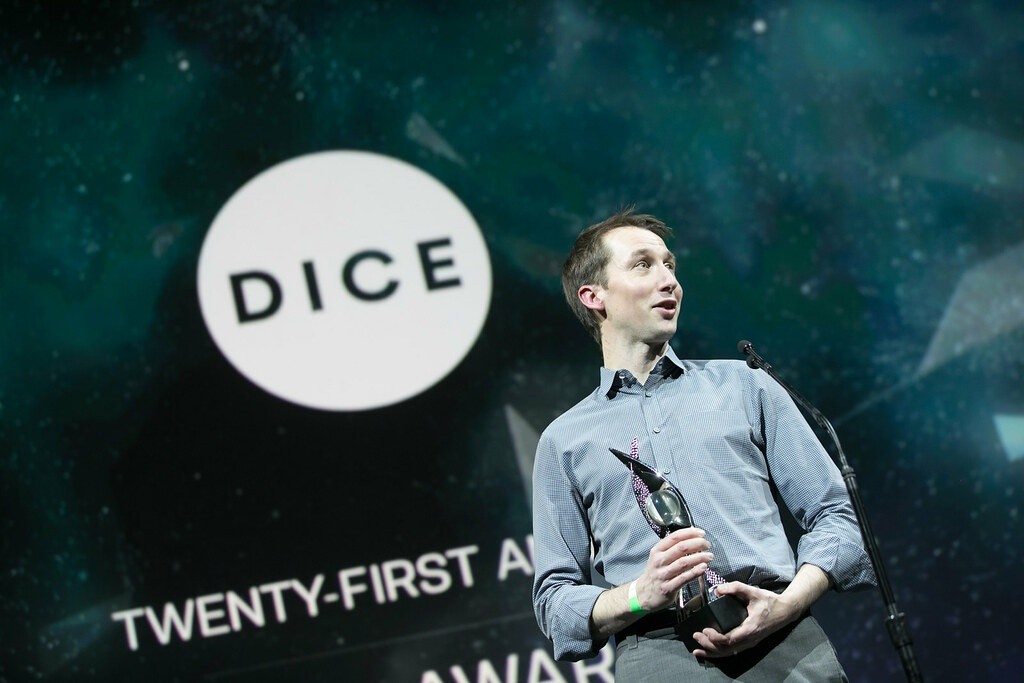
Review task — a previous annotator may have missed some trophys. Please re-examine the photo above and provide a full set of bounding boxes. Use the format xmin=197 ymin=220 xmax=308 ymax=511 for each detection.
xmin=609 ymin=447 xmax=750 ymax=652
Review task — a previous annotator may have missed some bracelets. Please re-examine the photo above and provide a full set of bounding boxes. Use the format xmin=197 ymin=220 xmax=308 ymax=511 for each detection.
xmin=628 ymin=580 xmax=648 ymax=616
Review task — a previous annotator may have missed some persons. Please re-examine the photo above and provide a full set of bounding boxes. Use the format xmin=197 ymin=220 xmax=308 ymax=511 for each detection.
xmin=531 ymin=203 xmax=878 ymax=683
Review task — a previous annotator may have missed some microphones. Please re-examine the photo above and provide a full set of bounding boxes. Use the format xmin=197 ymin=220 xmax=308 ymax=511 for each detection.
xmin=738 ymin=338 xmax=855 ymax=478
